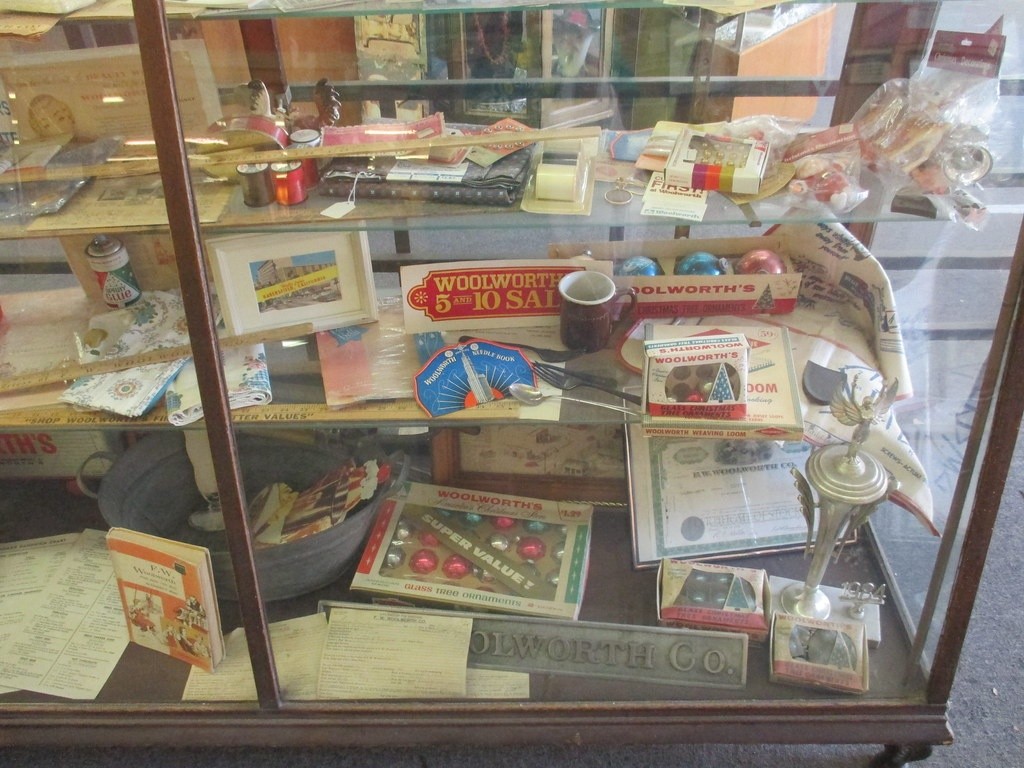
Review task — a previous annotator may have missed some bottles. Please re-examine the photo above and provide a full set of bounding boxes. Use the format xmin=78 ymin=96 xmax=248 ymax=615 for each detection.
xmin=84 ymin=233 xmax=142 ymax=311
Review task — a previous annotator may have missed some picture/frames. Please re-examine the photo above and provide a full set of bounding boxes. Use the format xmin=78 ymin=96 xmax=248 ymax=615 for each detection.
xmin=622 ymin=386 xmax=858 ymax=570
xmin=203 ymin=219 xmax=379 ymax=335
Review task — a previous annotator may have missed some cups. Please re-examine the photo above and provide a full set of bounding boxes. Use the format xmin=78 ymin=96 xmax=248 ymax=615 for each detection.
xmin=557 ymin=270 xmax=637 ymax=354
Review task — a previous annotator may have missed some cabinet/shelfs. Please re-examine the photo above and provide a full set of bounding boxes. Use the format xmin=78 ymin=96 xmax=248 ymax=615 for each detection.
xmin=0 ymin=0 xmax=1024 ymax=768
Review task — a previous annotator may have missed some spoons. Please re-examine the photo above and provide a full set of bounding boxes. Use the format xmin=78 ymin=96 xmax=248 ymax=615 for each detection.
xmin=509 ymin=383 xmax=641 ymax=419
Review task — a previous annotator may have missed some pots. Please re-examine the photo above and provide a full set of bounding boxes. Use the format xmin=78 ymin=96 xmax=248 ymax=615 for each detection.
xmin=76 ymin=431 xmax=411 ymax=601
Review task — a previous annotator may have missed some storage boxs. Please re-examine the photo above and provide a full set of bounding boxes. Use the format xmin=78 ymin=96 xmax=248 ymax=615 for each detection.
xmin=641 ymin=333 xmax=750 ymax=421
xmin=656 ymin=557 xmax=772 ymax=645
xmin=768 ymin=609 xmax=870 ymax=696
xmin=548 ymin=231 xmax=802 ymax=319
xmin=665 ymin=126 xmax=771 ymax=194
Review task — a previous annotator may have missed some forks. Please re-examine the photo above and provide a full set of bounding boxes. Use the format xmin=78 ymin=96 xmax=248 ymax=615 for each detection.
xmin=458 ymin=335 xmax=587 ymax=362
xmin=530 ymin=361 xmax=641 ymax=406
